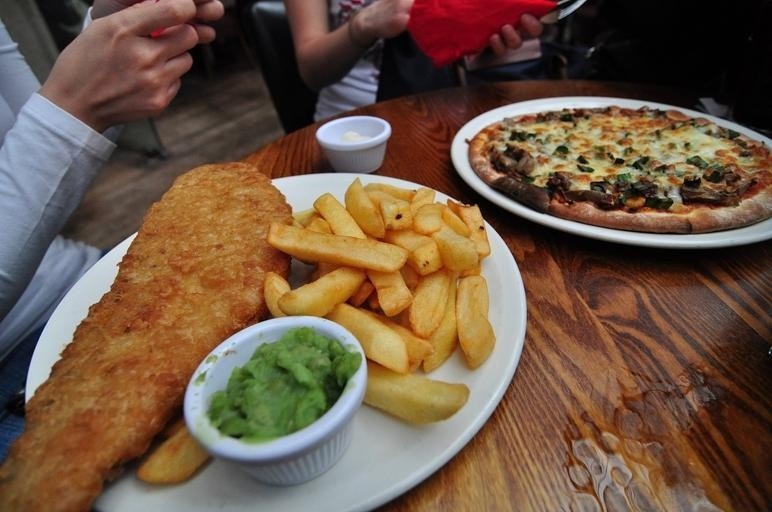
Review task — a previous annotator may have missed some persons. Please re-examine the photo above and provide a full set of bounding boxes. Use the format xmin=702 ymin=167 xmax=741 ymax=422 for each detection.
xmin=281 ymin=0 xmax=561 ymax=128
xmin=0 ymin=0 xmax=225 ymax=465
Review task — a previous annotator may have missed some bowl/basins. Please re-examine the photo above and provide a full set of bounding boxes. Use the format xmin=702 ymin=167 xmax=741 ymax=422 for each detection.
xmin=180 ymin=314 xmax=370 ymax=487
xmin=315 ymin=115 xmax=392 ymax=172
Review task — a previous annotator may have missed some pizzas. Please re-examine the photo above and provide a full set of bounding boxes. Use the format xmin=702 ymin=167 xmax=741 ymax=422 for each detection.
xmin=469 ymin=105 xmax=772 ymax=234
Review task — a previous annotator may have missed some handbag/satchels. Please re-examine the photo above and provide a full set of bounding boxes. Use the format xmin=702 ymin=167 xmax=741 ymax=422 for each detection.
xmin=406 ymin=0 xmax=556 ymax=66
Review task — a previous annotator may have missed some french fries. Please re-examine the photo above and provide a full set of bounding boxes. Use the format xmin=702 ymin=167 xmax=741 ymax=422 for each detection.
xmin=136 ymin=416 xmax=209 ymax=486
xmin=263 ymin=176 xmax=497 ymax=425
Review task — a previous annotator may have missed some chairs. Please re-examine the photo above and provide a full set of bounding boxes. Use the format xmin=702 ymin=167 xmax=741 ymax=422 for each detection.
xmin=249 ymin=1 xmax=319 ymax=136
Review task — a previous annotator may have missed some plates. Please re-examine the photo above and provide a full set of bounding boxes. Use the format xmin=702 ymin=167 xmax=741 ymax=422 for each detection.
xmin=450 ymin=94 xmax=772 ymax=247
xmin=23 ymin=172 xmax=528 ymax=512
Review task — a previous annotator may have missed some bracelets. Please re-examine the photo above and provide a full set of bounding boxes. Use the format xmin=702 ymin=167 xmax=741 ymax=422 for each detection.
xmin=348 ymin=7 xmax=378 ymax=48
